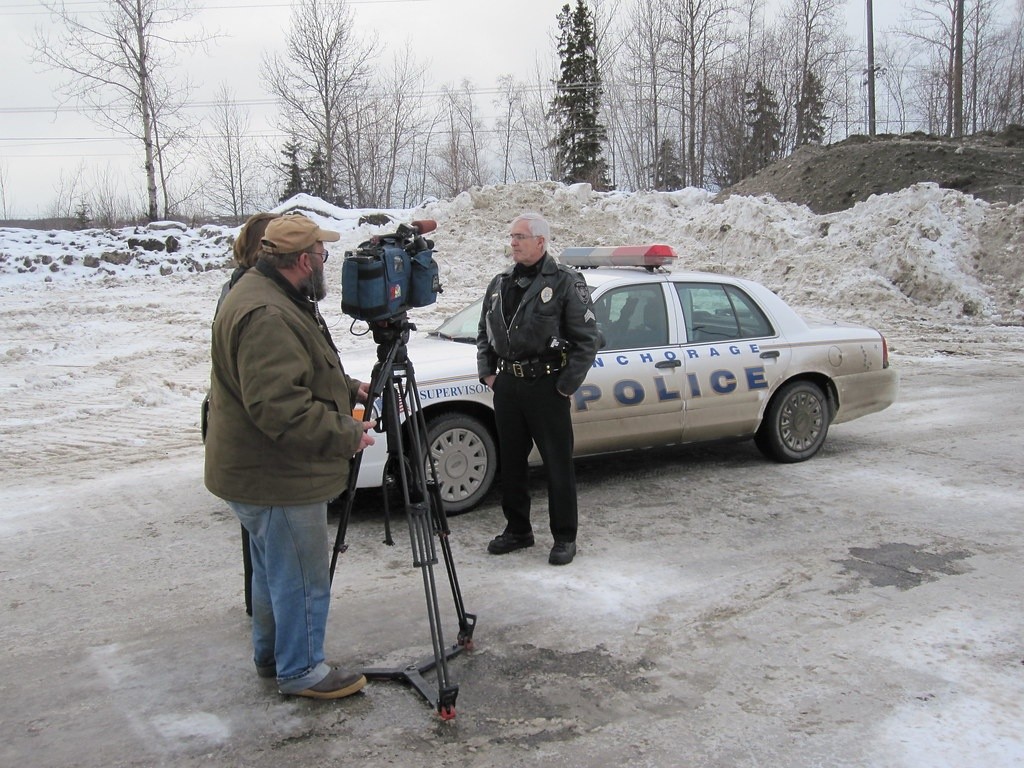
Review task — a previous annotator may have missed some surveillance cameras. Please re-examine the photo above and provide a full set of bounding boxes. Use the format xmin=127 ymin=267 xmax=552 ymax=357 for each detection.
xmin=344 ymin=235 xmax=442 ymax=318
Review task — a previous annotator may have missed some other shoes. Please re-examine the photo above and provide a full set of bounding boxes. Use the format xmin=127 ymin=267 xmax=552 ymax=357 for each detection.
xmin=293 ymin=669 xmax=367 ymax=699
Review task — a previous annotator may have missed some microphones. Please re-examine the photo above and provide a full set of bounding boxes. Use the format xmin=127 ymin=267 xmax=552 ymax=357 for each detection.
xmin=412 ymin=220 xmax=437 ymax=234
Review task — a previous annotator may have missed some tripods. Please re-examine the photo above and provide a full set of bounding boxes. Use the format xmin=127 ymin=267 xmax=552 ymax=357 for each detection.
xmin=325 ymin=321 xmax=479 ymax=716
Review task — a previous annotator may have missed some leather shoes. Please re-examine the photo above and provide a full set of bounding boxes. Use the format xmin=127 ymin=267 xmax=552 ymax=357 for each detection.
xmin=548 ymin=540 xmax=576 ymax=566
xmin=487 ymin=526 xmax=535 ymax=554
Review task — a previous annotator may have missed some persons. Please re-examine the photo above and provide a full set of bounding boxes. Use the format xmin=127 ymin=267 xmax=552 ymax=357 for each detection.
xmin=212 ymin=211 xmax=282 ymax=617
xmin=474 ymin=211 xmax=602 ymax=567
xmin=203 ymin=211 xmax=378 ymax=699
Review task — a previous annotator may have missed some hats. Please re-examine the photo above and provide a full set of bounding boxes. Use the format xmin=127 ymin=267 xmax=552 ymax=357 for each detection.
xmin=261 ymin=213 xmax=340 ymax=253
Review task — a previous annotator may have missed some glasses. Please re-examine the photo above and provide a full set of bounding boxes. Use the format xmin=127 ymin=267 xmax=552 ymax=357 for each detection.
xmin=510 ymin=234 xmax=538 ymax=239
xmin=309 ymin=249 xmax=329 ymax=263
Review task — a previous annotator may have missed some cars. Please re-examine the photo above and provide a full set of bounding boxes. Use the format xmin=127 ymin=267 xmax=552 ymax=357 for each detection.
xmin=341 ymin=243 xmax=900 ymax=517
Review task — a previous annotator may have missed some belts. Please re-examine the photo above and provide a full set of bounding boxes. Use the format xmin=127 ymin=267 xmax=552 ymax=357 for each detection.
xmin=497 ymin=354 xmax=568 ymax=378
